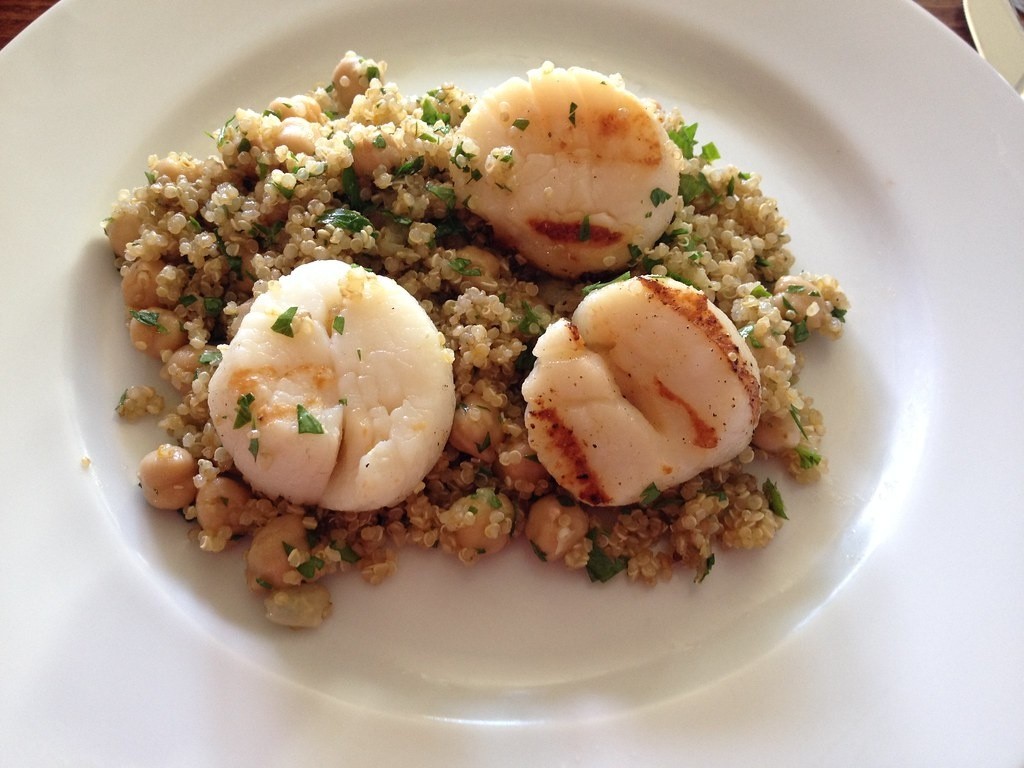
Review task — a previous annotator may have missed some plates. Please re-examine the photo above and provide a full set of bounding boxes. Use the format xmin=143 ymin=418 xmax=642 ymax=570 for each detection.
xmin=0 ymin=1 xmax=1024 ymax=767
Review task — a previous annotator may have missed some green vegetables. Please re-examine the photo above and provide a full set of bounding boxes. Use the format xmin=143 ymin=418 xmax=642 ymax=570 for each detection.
xmin=103 ymin=58 xmax=850 ymax=584
